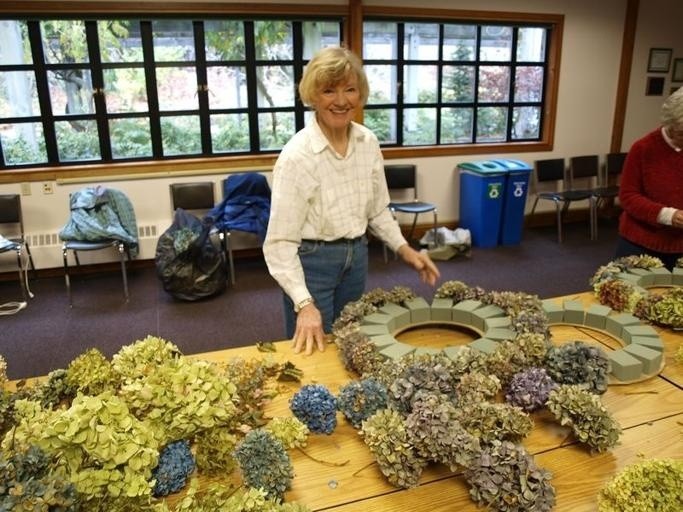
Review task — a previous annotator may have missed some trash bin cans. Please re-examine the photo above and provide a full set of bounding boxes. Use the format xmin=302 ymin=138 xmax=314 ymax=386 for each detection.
xmin=455 ymin=159 xmax=534 ymax=247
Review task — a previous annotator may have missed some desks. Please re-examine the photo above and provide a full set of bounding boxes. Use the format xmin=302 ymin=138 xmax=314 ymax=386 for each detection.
xmin=0 ymin=288 xmax=683 ymax=512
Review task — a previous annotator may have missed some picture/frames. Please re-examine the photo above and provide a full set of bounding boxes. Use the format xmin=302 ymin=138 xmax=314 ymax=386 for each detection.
xmin=648 ymin=47 xmax=673 ymax=73
xmin=670 ymin=87 xmax=680 ymax=94
xmin=644 ymin=76 xmax=665 ymax=96
xmin=672 ymin=58 xmax=683 ymax=82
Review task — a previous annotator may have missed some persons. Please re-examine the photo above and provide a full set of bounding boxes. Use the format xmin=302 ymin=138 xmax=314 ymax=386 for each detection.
xmin=613 ymin=88 xmax=682 ymax=275
xmin=259 ymin=43 xmax=445 ymax=360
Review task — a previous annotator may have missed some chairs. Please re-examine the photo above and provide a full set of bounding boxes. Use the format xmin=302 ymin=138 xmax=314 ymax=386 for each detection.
xmin=168 ymin=181 xmax=225 ymax=248
xmin=0 ymin=194 xmax=38 ymax=300
xmin=532 ymin=153 xmax=627 ymax=244
xmin=62 ymin=193 xmax=131 ymax=308
xmin=221 ymin=173 xmax=271 ymax=285
xmin=382 ymin=165 xmax=438 ymax=260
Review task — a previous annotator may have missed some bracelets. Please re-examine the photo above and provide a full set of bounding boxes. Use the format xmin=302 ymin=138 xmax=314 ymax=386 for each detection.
xmin=293 ymin=297 xmax=313 ymax=312
